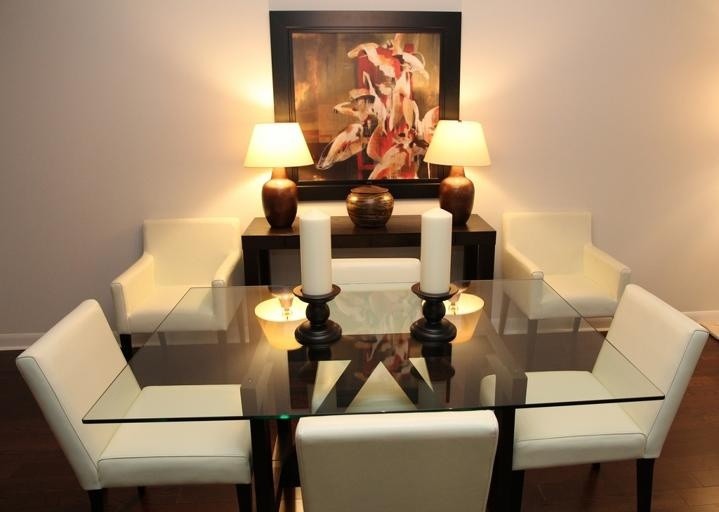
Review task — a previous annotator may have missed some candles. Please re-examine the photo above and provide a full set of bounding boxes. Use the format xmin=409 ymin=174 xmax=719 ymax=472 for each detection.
xmin=299 ymin=209 xmax=333 ymax=297
xmin=420 ymin=208 xmax=453 ymax=295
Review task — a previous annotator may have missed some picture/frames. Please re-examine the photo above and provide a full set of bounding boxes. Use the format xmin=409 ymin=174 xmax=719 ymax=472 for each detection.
xmin=267 ymin=10 xmax=462 ymax=200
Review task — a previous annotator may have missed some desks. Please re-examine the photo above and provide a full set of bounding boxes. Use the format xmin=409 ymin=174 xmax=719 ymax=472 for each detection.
xmin=81 ymin=277 xmax=669 ymax=512
xmin=240 ymin=214 xmax=497 ymax=322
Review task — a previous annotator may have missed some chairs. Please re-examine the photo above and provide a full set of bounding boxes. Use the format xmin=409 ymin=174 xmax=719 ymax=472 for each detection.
xmin=497 ymin=209 xmax=629 ymax=367
xmin=478 ymin=283 xmax=709 ymax=512
xmin=14 ymin=298 xmax=284 ymax=512
xmin=309 ymin=255 xmax=434 ymax=412
xmin=110 ymin=214 xmax=247 ymax=384
xmin=294 ymin=410 xmax=499 ymax=512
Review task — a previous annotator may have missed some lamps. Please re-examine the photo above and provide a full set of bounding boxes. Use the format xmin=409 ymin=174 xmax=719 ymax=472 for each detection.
xmin=243 ymin=119 xmax=315 ymax=229
xmin=255 ymin=286 xmax=309 ymax=353
xmin=421 ymin=287 xmax=485 ymax=345
xmin=422 ymin=116 xmax=492 ymax=228
